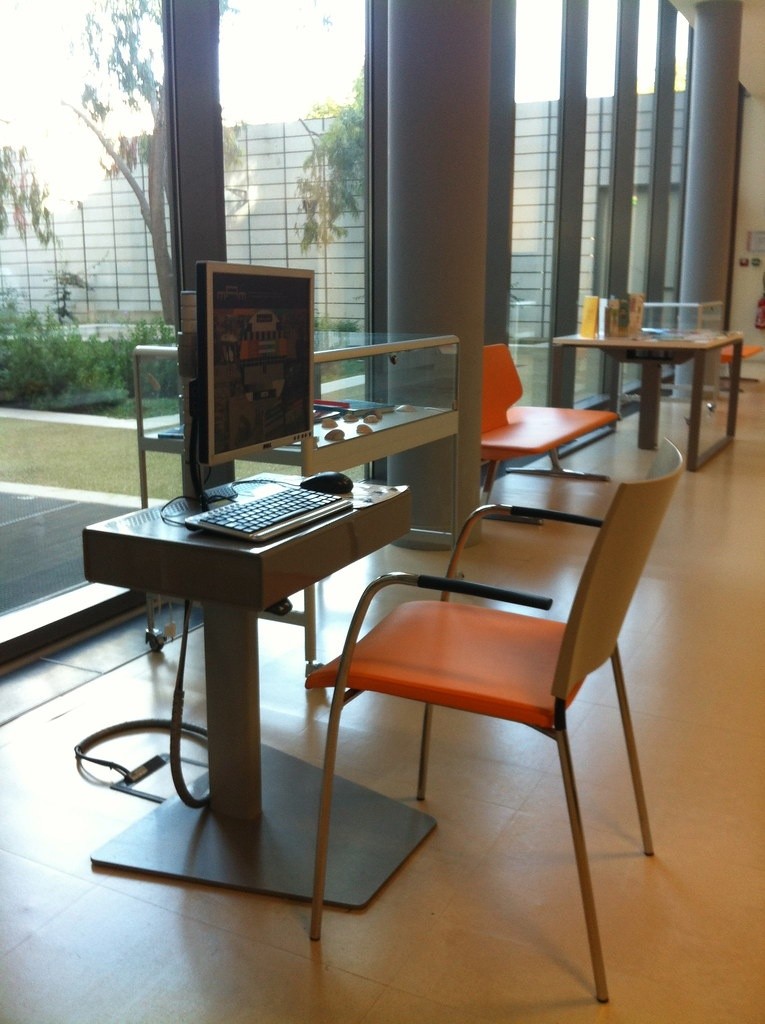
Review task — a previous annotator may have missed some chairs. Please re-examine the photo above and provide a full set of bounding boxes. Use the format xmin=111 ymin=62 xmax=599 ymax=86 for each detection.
xmin=309 ymin=436 xmax=684 ymax=1005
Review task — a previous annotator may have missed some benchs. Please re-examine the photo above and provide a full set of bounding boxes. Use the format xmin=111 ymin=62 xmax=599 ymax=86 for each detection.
xmin=480 ymin=343 xmax=610 ymax=526
xmin=721 ymin=346 xmax=765 ymax=393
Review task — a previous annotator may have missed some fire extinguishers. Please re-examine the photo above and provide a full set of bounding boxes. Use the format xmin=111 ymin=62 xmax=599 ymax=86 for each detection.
xmin=755 ymin=292 xmax=765 ymax=330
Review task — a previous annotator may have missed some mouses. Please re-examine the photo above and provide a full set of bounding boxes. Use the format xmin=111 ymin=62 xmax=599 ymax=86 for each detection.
xmin=300 ymin=471 xmax=353 ymax=495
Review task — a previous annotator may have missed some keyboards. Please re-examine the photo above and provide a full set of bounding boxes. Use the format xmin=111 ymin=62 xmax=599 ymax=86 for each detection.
xmin=185 ymin=485 xmax=353 ymax=541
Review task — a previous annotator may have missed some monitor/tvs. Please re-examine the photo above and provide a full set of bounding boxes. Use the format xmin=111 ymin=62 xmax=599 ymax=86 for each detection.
xmin=190 ymin=259 xmax=314 ymax=466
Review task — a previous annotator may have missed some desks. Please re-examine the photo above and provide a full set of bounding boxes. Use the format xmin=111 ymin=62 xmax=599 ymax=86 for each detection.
xmin=553 ymin=327 xmax=743 ymax=473
xmin=82 ymin=473 xmax=438 ymax=912
